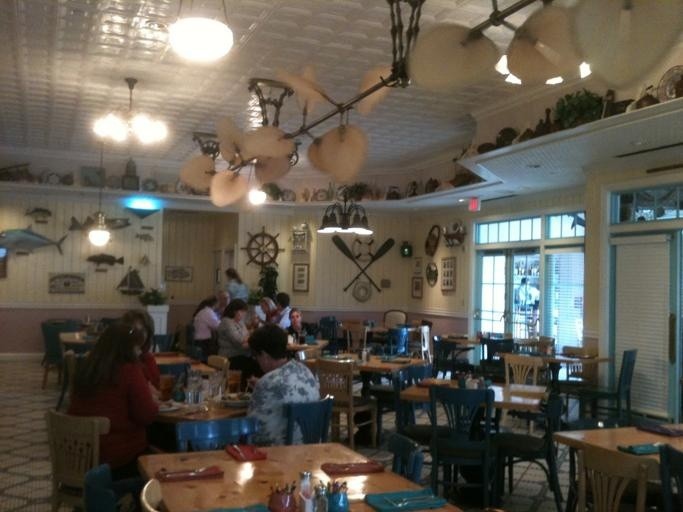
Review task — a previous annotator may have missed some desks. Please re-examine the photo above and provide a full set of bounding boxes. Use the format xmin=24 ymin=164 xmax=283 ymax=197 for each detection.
xmin=136 ymin=442 xmax=463 ymax=511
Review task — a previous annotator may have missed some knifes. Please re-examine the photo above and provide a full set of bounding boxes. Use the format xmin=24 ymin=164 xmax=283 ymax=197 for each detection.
xmin=231 ymin=444 xmax=245 ymax=460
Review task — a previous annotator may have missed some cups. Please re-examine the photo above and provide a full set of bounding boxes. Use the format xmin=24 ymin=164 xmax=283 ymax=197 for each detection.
xmin=297 ymin=331 xmax=305 ymax=344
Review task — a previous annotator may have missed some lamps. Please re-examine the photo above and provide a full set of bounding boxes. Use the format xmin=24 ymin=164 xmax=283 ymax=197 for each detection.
xmin=92 ymin=77 xmax=168 ymax=147
xmin=165 ymin=1 xmax=236 ymax=66
xmin=316 ymin=188 xmax=374 ymax=237
xmin=88 ymin=144 xmax=111 ymax=248
xmin=177 ymin=63 xmax=400 ymax=208
xmin=404 ymin=1 xmax=682 ymax=92
xmin=125 ymin=207 xmax=160 ymax=220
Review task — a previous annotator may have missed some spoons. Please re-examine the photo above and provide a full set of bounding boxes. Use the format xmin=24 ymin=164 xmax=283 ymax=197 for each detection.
xmin=161 ymin=462 xmax=212 ymax=475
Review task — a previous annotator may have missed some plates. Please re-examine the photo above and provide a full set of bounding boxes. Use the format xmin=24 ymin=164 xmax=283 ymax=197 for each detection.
xmin=144 ymin=179 xmax=159 ymax=192
xmin=281 ymin=189 xmax=296 ymax=201
xmin=216 ymin=391 xmax=251 ymax=408
xmin=175 ymin=180 xmax=190 ymax=194
xmin=159 ymin=401 xmax=181 ymax=412
xmin=336 ymin=186 xmax=345 ymax=201
xmin=316 ymin=189 xmax=328 ymax=201
xmin=658 ymin=66 xmax=682 ymax=102
xmin=496 ymin=127 xmax=515 ymax=145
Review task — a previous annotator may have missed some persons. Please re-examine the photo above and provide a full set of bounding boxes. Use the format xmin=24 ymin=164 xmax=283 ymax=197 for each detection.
xmin=66 ymin=306 xmax=164 ymax=465
xmin=244 ymin=323 xmax=321 ymax=447
xmin=190 ymin=292 xmax=312 ymax=383
xmin=224 ymin=268 xmax=249 ymax=302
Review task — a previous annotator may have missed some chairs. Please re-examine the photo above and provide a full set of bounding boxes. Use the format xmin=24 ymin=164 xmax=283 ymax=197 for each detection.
xmin=390 ymin=372 xmax=446 ymax=472
xmin=538 ymin=411 xmax=682 ymax=512
xmin=173 ymin=415 xmax=257 ymax=452
xmin=51 ymin=309 xmax=643 ymax=424
xmin=495 ymin=379 xmax=563 ymax=510
xmin=425 ymin=382 xmax=513 ymax=512
xmin=383 ymin=432 xmax=426 ymax=486
xmin=136 ymin=476 xmax=164 ymax=512
xmin=38 ymin=409 xmax=112 ymax=512
xmin=281 ymin=397 xmax=336 ymax=445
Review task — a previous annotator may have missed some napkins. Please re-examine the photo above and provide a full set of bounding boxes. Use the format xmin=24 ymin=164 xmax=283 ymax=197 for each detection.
xmin=222 ymin=443 xmax=271 ymax=463
xmin=367 ymin=488 xmax=447 ymax=512
xmin=156 ymin=465 xmax=227 ymax=485
xmin=320 ymin=460 xmax=384 ymax=481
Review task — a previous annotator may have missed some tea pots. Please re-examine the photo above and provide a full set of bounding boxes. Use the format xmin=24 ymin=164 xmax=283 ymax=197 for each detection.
xmin=635 ymin=85 xmax=659 ymax=109
xmin=386 ymin=186 xmax=400 ymax=199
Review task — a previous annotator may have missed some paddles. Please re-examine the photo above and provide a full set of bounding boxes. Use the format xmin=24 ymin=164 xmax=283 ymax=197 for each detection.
xmin=344 ymin=238 xmax=395 ymax=291
xmin=332 ymin=235 xmax=381 ymax=292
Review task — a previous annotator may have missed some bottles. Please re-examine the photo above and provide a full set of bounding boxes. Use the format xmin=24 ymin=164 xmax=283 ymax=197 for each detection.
xmin=361 ymin=348 xmax=369 ymax=360
xmin=327 ymin=181 xmax=336 ymax=201
xmin=309 ymin=185 xmax=316 ymax=202
xmin=263 ymin=470 xmax=350 ymax=512
xmin=455 ymin=370 xmax=485 ymax=390
xmin=183 ymin=372 xmax=222 ymax=410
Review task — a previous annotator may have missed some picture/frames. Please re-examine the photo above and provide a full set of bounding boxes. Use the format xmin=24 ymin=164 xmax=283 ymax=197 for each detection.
xmin=293 ymin=263 xmax=309 ymax=292
xmin=411 ymin=277 xmax=423 ymax=299
xmin=441 ymin=257 xmax=456 ymax=291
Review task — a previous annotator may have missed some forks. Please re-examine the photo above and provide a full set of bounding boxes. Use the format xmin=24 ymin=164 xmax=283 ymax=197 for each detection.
xmin=382 ymin=495 xmax=437 ymax=505
